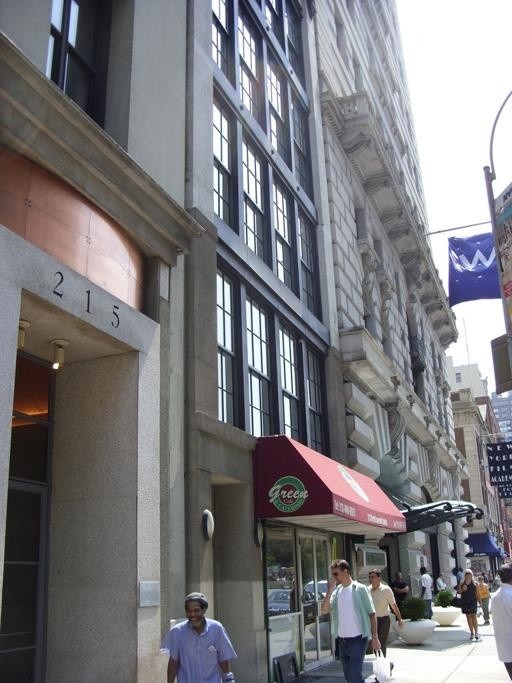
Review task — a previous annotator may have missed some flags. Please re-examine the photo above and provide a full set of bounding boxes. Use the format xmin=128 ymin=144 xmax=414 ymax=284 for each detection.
xmin=447 ymin=234 xmax=503 ymax=309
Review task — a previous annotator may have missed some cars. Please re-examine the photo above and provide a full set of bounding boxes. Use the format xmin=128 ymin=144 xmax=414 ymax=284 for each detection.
xmin=266 ymin=585 xmax=327 ymax=627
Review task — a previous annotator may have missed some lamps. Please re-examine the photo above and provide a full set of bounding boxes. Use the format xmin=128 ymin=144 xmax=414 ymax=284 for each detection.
xmin=46 ymin=338 xmax=72 ymax=371
xmin=16 ymin=319 xmax=32 ymax=351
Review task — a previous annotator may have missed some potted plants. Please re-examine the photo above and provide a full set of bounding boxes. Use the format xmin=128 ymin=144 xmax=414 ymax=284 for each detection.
xmin=391 ymin=596 xmax=440 ymax=645
xmin=433 ymin=590 xmax=463 ymax=627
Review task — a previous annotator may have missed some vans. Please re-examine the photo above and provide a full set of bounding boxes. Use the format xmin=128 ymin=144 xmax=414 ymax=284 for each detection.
xmin=303 ymin=579 xmax=330 ymax=603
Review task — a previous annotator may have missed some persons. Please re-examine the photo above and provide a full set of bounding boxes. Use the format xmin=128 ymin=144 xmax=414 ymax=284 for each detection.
xmin=490 ymin=561 xmax=512 ymax=679
xmin=321 ymin=559 xmax=381 ymax=682
xmin=390 ymin=565 xmax=503 ymax=626
xmin=457 ymin=568 xmax=484 ymax=639
xmin=158 ymin=592 xmax=238 ymax=682
xmin=367 ymin=567 xmax=403 ymax=676
